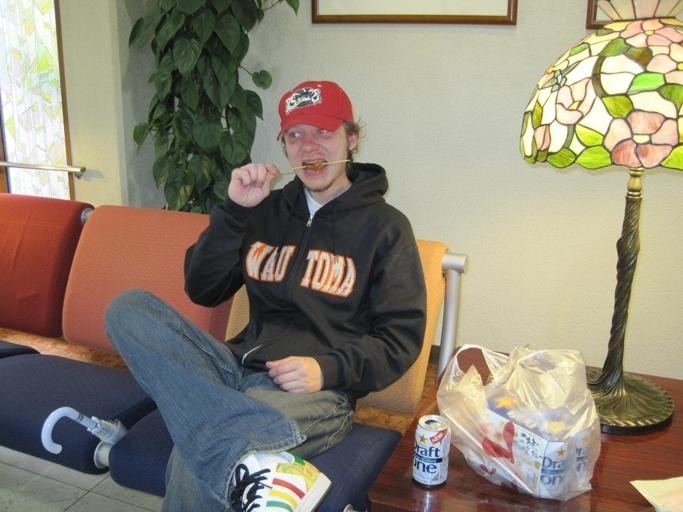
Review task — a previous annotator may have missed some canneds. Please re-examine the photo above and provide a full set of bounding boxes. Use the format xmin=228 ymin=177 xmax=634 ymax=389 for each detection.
xmin=411 ymin=414 xmax=452 ymax=489
xmin=570 ymin=428 xmax=592 ymax=491
xmin=541 ymin=442 xmax=570 ymax=496
xmin=411 ymin=482 xmax=443 ymax=512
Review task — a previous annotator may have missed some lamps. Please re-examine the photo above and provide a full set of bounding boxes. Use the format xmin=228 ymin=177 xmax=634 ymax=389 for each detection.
xmin=520 ymin=1 xmax=683 ymax=436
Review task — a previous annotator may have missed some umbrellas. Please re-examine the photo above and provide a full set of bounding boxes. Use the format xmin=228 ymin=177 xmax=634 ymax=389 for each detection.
xmin=40 ymin=405 xmax=128 ymax=455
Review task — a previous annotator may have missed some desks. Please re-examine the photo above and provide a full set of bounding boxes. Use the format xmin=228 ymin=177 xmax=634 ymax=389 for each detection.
xmin=367 ymin=344 xmax=683 ymax=512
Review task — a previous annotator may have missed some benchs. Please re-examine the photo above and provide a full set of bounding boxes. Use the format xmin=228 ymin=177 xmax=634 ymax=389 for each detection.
xmin=0 ymin=189 xmax=467 ymax=512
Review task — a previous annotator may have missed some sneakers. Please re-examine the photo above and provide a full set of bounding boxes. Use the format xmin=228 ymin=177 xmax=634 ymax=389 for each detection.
xmin=227 ymin=450 xmax=332 ymax=512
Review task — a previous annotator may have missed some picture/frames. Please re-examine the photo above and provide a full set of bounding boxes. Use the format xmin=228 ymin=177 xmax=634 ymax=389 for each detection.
xmin=586 ymin=1 xmax=612 ymax=29
xmin=311 ymin=0 xmax=517 ymax=26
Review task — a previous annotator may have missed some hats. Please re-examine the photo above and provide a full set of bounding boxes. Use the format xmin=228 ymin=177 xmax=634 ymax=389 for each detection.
xmin=276 ymin=80 xmax=353 ymax=142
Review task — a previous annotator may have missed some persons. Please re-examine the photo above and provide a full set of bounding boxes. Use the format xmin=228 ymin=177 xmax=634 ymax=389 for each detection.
xmin=103 ymin=80 xmax=426 ymax=511
